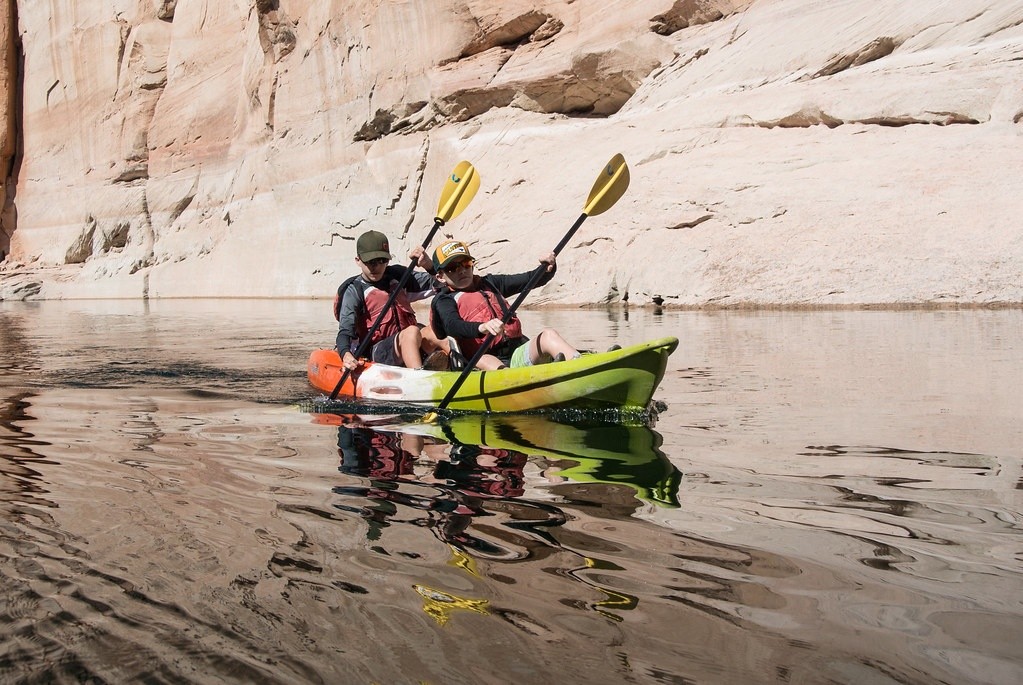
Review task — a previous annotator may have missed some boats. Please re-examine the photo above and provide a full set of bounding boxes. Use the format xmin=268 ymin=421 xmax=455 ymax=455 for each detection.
xmin=304 ymin=336 xmax=682 ymax=415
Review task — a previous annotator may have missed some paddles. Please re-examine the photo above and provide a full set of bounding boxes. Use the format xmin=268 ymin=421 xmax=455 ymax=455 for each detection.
xmin=421 ymin=152 xmax=632 ymax=426
xmin=328 ymin=158 xmax=483 ymax=401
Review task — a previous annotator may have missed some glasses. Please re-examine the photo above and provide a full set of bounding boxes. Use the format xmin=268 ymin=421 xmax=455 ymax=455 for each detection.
xmin=367 ymin=258 xmax=389 ymax=264
xmin=443 ymin=258 xmax=474 ymax=273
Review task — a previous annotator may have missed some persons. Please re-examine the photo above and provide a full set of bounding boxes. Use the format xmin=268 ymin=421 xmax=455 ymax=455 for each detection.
xmin=336 ymin=230 xmax=452 ymax=370
xmin=432 ymin=240 xmax=622 ymax=371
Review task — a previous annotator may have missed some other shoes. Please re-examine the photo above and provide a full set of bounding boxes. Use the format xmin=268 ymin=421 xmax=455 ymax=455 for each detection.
xmin=423 ymin=350 xmax=449 ymax=371
xmin=554 ymin=352 xmax=566 ymax=362
xmin=607 ymin=345 xmax=621 ymax=352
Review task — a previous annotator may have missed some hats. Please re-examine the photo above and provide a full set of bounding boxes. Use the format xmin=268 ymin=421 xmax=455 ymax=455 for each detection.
xmin=357 ymin=230 xmax=392 ymax=263
xmin=433 ymin=240 xmax=475 ymax=273
